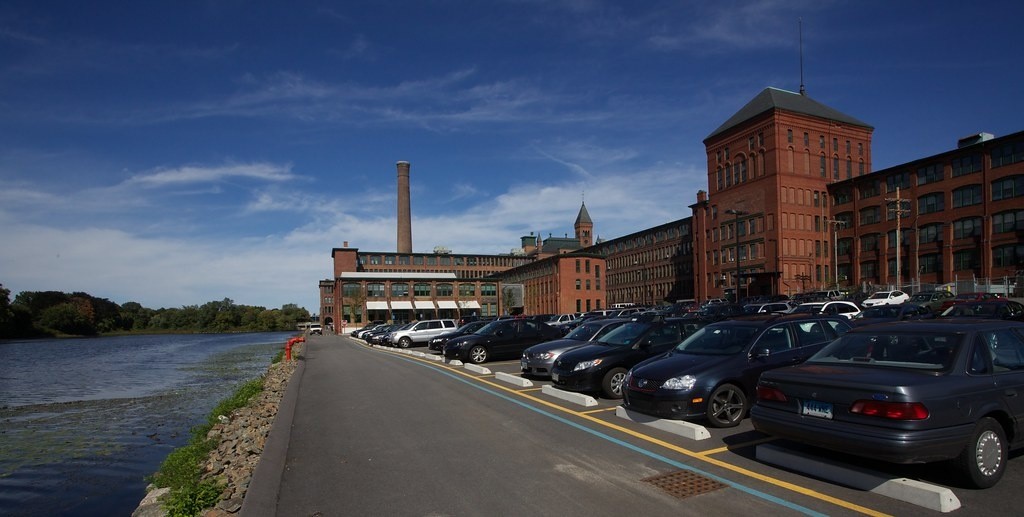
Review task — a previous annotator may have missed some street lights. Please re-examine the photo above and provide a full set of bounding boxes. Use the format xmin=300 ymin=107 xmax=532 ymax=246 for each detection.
xmin=726 ymin=210 xmax=742 ymax=303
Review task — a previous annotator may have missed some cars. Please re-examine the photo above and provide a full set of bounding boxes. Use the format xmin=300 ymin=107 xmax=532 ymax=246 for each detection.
xmin=749 ymin=318 xmax=1024 ymax=489
xmin=428 ymin=321 xmax=514 ymax=350
xmin=834 ymin=303 xmax=935 ymax=350
xmin=520 ymin=320 xmax=638 ymax=376
xmin=861 ymin=290 xmax=910 ymax=308
xmin=939 ymin=301 xmax=1024 ymax=350
xmin=551 ymin=318 xmax=722 ymax=399
xmin=621 ymin=313 xmax=859 ymax=428
xmin=351 ymin=321 xmax=425 ymax=347
xmin=902 ymin=290 xmax=956 ymax=314
xmin=310 ymin=324 xmax=323 ymax=335
xmin=533 ymin=290 xmax=863 ymax=332
xmin=442 ymin=319 xmax=562 ymax=364
xmin=937 ymin=292 xmax=1008 ymax=316
xmin=391 ymin=319 xmax=459 ymax=348
